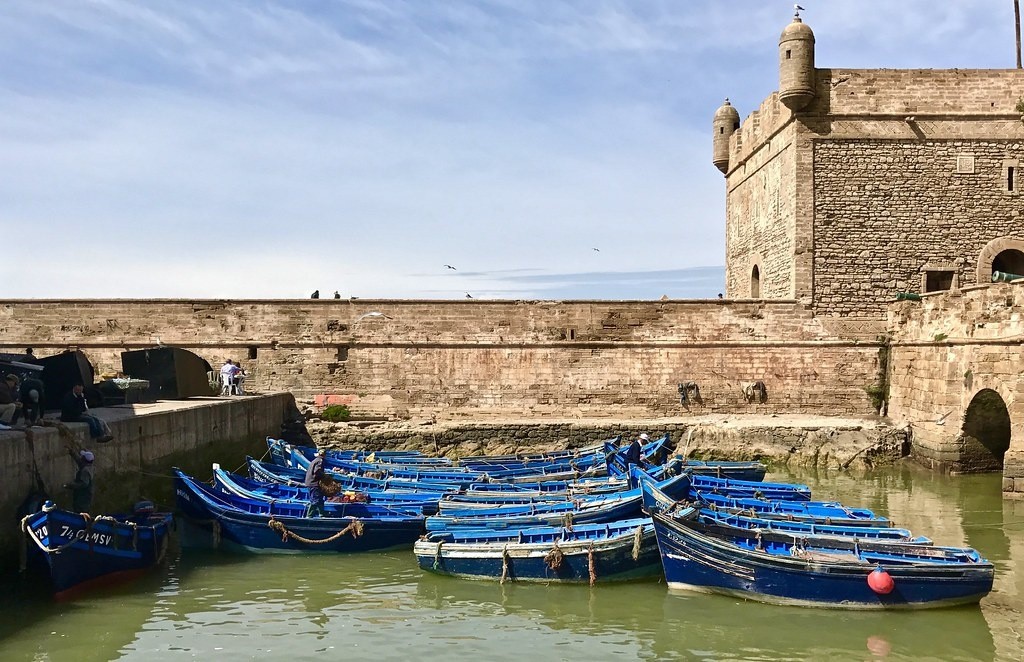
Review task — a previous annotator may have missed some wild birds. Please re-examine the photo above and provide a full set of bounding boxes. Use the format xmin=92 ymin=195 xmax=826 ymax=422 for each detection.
xmin=444 ymin=264 xmax=456 ymax=270
xmin=334 ymin=290 xmax=340 ymax=299
xmin=351 ymin=294 xmax=359 ymax=299
xmin=354 ymin=312 xmax=393 ymax=324
xmin=591 ymin=248 xmax=599 ymax=251
xmin=465 ymin=291 xmax=473 ymax=299
xmin=155 ymin=336 xmax=164 ymax=344
xmin=718 ymin=292 xmax=723 ymax=299
xmin=310 ymin=289 xmax=319 ymax=299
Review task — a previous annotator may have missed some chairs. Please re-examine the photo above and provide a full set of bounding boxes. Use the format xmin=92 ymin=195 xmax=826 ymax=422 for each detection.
xmin=207 ymin=370 xmax=244 ymax=396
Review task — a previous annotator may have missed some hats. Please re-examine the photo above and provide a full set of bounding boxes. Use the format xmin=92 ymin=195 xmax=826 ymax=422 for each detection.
xmin=7 ymin=374 xmax=19 ymax=386
xmin=29 ymin=390 xmax=39 ymax=403
xmin=80 ymin=450 xmax=95 ymax=462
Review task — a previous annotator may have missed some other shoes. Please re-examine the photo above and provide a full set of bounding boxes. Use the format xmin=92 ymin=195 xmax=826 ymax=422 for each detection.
xmin=30 ymin=420 xmax=36 ymax=426
xmin=0 ymin=424 xmax=12 ymax=430
xmin=97 ymin=435 xmax=114 ymax=444
xmin=10 ymin=421 xmax=17 ymax=425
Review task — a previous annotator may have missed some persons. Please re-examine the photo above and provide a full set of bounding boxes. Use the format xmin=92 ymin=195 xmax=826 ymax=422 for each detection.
xmin=60 ymin=383 xmax=114 ymax=443
xmin=305 ymin=449 xmax=329 ymax=519
xmin=62 ymin=445 xmax=98 ymax=517
xmin=624 ymin=433 xmax=651 ymax=472
xmin=333 ymin=290 xmax=341 ymax=299
xmin=17 ymin=348 xmax=37 ymax=379
xmin=220 ymin=359 xmax=246 ymax=395
xmin=0 ymin=373 xmax=45 ymax=430
xmin=311 ymin=289 xmax=320 ymax=299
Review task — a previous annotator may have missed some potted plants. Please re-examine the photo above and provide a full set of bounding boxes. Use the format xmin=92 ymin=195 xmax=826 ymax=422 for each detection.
xmin=207 ymin=381 xmax=220 ymax=396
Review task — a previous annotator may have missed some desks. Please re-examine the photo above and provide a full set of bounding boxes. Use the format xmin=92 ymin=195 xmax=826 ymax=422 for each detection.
xmin=233 ymin=375 xmax=244 ymax=379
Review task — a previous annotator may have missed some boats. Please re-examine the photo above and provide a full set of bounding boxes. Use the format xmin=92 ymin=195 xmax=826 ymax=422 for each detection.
xmin=425 ymin=465 xmax=694 ymax=532
xmin=446 ymin=453 xmax=684 ymax=516
xmin=266 ymin=436 xmax=469 ymax=474
xmin=24 ymin=498 xmax=173 ymax=602
xmin=245 ymin=454 xmax=462 ymax=497
xmin=211 ymin=462 xmax=445 ymax=516
xmin=171 ymin=466 xmax=429 ymax=555
xmin=414 ymin=501 xmax=705 ymax=581
xmin=289 ymin=445 xmax=474 ymax=490
xmin=608 ymin=461 xmax=812 ymax=501
xmin=629 ymin=462 xmax=894 ymax=527
xmin=458 ymin=432 xmax=670 ymax=495
xmin=636 ymin=475 xmax=934 ymax=547
xmin=648 ymin=505 xmax=995 ymax=608
xmin=606 ymin=441 xmax=767 ymax=482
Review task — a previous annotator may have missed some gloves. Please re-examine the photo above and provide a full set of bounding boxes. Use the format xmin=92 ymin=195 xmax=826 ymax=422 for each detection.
xmin=38 ymin=418 xmax=43 ymax=425
xmin=24 ymin=419 xmax=31 ymax=425
